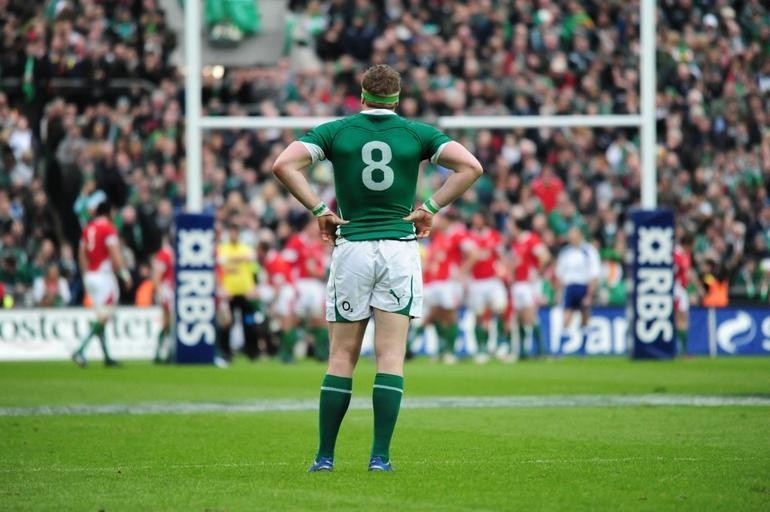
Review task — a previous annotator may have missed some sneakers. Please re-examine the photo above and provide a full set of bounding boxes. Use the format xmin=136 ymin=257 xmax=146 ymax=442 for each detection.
xmin=368 ymin=458 xmax=393 ymax=472
xmin=309 ymin=455 xmax=336 ymax=472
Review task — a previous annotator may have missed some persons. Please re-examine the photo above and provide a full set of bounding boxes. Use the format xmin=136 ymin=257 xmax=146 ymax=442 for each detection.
xmin=1 ymin=1 xmax=769 ymax=364
xmin=273 ymin=65 xmax=483 ymax=470
